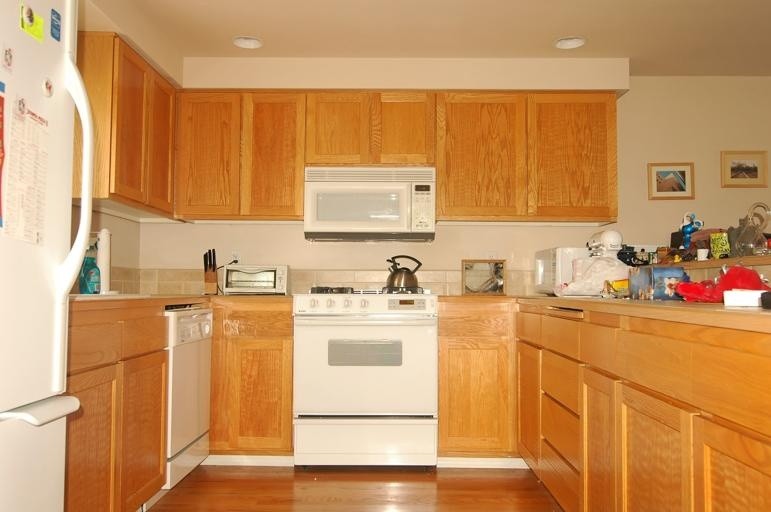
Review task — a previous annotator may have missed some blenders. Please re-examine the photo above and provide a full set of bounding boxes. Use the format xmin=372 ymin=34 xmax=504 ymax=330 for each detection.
xmin=587 ymin=229 xmax=622 ymax=259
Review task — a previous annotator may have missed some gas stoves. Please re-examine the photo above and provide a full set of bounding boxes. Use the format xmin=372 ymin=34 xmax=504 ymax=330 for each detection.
xmin=291 ymin=286 xmax=438 ymax=314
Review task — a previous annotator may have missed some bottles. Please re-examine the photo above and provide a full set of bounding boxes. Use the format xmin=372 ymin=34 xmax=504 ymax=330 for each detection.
xmin=735 ymin=216 xmax=769 ymax=257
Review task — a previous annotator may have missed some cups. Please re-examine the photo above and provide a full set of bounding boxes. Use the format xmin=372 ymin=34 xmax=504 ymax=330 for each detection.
xmin=762 ymin=292 xmax=771 ymax=308
xmin=710 ymin=232 xmax=728 ymax=259
xmin=695 ymin=249 xmax=708 ymax=260
xmin=635 ymin=249 xmax=649 ymax=264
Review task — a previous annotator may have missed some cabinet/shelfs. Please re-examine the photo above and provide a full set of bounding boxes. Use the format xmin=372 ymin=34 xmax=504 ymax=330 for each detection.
xmin=72 ymin=32 xmax=175 ymax=223
xmin=516 ymin=298 xmax=542 ymax=481
xmin=173 ymin=88 xmax=306 ymax=225
xmin=543 ymin=299 xmax=583 ymax=512
xmin=583 ymin=300 xmax=621 ymax=512
xmin=436 ymin=90 xmax=618 ymax=228
xmin=623 ymin=301 xmax=701 ymax=512
xmin=208 ymin=296 xmax=294 ymax=467
xmin=65 ymin=298 xmax=170 ymax=512
xmin=692 ymin=307 xmax=771 ymax=512
xmin=437 ymin=295 xmax=519 ymax=468
xmin=307 ymin=88 xmax=435 ymax=164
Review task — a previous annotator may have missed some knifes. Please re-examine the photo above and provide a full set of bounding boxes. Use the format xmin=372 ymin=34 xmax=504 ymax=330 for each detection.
xmin=204 ymin=249 xmax=217 ymax=272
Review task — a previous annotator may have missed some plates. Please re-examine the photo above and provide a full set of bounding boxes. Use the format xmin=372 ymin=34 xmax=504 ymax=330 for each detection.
xmin=611 ymin=292 xmax=629 ymax=298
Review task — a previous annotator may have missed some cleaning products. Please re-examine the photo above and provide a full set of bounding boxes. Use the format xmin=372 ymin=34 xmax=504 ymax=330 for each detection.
xmin=78 ymin=259 xmax=101 ymax=294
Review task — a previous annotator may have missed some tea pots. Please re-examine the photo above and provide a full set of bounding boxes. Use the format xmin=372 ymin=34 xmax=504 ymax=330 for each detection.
xmin=385 ymin=255 xmax=422 ymax=291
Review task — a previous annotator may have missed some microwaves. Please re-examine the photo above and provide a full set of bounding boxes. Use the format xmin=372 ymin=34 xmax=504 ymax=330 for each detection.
xmin=534 ymin=248 xmax=589 ymax=298
xmin=224 ymin=264 xmax=287 ymax=296
xmin=304 ymin=167 xmax=434 ymax=234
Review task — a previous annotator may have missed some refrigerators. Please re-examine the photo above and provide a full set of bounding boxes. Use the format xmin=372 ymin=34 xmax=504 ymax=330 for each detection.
xmin=2 ymin=0 xmax=95 ymax=510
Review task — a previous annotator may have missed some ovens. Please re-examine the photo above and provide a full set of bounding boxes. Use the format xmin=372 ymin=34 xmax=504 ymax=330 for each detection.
xmin=292 ymin=318 xmax=438 ymax=418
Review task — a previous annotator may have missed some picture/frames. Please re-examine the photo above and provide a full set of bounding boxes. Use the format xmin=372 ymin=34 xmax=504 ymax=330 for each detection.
xmin=720 ymin=150 xmax=769 ymax=188
xmin=461 ymin=259 xmax=508 ymax=296
xmin=647 ymin=162 xmax=696 ymax=201
